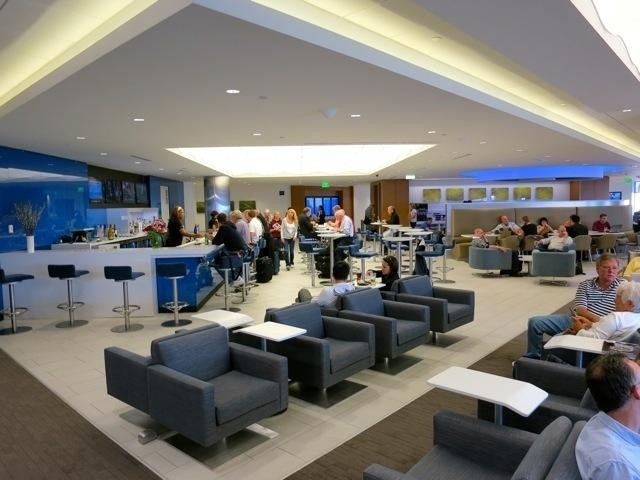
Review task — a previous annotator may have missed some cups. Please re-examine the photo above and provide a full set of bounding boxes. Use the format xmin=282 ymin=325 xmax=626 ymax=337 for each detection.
xmin=604 ymin=227 xmax=608 ymax=232
xmin=8 ymin=224 xmax=13 ymax=234
xmin=324 ymin=223 xmax=328 ymax=227
xmin=382 ymin=219 xmax=385 ymax=223
xmin=522 ymin=198 xmax=526 ymax=201
xmin=483 ymin=198 xmax=487 ymax=201
xmin=491 ymin=195 xmax=496 ymax=200
xmin=377 ymin=219 xmax=380 ymax=223
xmin=518 ymin=250 xmax=525 ymax=259
xmin=370 ymin=273 xmax=376 ymax=286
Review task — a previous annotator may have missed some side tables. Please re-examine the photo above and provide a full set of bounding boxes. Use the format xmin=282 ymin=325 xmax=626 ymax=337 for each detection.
xmin=351 ymin=273 xmax=412 ymax=291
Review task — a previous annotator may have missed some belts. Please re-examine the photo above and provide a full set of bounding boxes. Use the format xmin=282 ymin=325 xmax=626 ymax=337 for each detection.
xmin=551 ymin=248 xmax=562 ymax=252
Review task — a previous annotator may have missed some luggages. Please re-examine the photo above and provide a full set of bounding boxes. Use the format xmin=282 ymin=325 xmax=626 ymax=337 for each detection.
xmin=269 ymin=238 xmax=279 ymax=275
xmin=256 ymin=235 xmax=273 ymax=283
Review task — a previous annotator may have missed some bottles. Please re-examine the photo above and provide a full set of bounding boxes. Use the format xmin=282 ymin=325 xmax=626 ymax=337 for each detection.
xmin=129 ymin=216 xmax=155 ymax=236
xmin=108 ymin=224 xmax=114 ymax=239
xmin=194 ymin=224 xmax=209 ymax=245
xmin=356 ymin=268 xmax=361 ymax=283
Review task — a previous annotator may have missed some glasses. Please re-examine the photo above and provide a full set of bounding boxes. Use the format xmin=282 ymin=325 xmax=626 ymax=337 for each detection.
xmin=384 ymin=255 xmax=394 ymax=264
xmin=596 ymin=266 xmax=619 ymax=270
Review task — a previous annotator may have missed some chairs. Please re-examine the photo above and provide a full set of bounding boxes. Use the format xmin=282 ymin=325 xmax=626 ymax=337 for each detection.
xmin=0 ymin=269 xmax=35 ymax=335
xmin=450 ymin=205 xmax=634 ymax=286
xmin=48 ymin=264 xmax=90 ymax=328
xmin=213 ymin=260 xmax=255 ymax=312
xmin=104 ymin=265 xmax=145 ymax=332
xmin=297 ymin=219 xmax=458 ymax=286
xmin=158 ymin=263 xmax=192 ymax=327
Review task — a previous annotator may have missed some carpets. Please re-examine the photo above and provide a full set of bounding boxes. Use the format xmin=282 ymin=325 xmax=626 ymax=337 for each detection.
xmin=0 ymin=347 xmax=164 ymax=478
xmin=285 ymin=294 xmax=640 ymax=480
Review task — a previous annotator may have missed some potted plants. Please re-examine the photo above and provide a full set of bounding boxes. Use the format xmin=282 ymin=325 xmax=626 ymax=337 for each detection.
xmin=11 ymin=200 xmax=46 ymax=253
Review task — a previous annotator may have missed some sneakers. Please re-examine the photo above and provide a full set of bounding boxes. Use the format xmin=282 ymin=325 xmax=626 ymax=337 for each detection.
xmin=509 ymin=272 xmax=524 ymax=277
xmin=286 ymin=265 xmax=290 ymax=271
xmin=295 ymin=298 xmax=299 ymax=303
xmin=228 ymin=278 xmax=243 ymax=290
xmin=512 ymin=353 xmax=541 ymax=367
xmin=341 ymin=254 xmax=347 ymax=261
xmin=290 ymin=264 xmax=294 ymax=268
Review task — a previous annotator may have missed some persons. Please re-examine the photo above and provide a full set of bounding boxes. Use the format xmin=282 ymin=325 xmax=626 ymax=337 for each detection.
xmin=623 ymin=255 xmax=640 ymax=283
xmin=165 ymin=205 xmax=202 ymax=247
xmin=472 ymin=214 xmax=610 ymax=276
xmin=208 ymin=204 xmax=446 ymax=310
xmin=575 ymin=350 xmax=640 ymax=479
xmin=557 ymin=282 xmax=640 ymax=364
xmin=527 ymin=279 xmax=640 ymax=356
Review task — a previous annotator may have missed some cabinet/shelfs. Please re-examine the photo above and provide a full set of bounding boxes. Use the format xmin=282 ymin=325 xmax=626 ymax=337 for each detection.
xmin=370 ymin=179 xmax=409 ymax=227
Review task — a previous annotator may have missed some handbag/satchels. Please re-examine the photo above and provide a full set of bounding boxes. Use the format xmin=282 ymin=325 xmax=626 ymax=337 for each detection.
xmin=222 ymin=250 xmax=243 ymax=267
xmin=278 ymin=238 xmax=284 ymax=248
xmin=576 ymin=260 xmax=582 ymax=274
xmin=363 ymin=216 xmax=371 ymax=225
